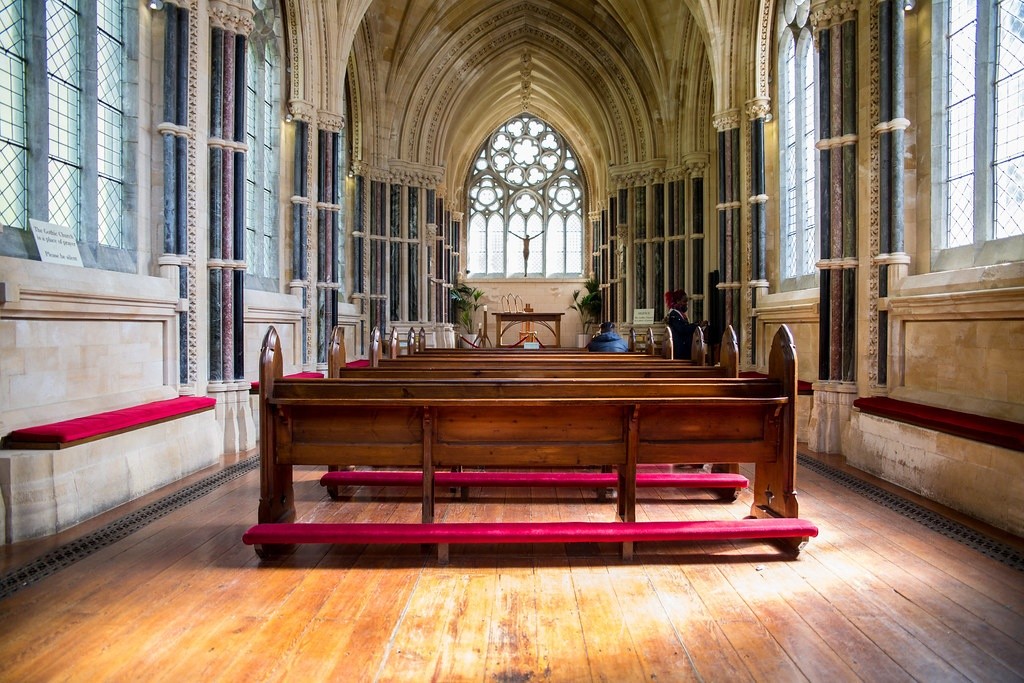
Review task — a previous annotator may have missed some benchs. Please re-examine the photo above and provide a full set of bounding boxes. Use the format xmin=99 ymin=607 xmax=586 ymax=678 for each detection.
xmin=243 ymin=325 xmax=819 ymax=563
xmin=853 ymin=396 xmax=1024 ymax=453
xmin=4 ymin=397 xmax=217 ymax=450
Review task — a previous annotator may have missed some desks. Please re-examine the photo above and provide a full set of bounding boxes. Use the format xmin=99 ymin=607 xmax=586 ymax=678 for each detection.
xmin=491 ymin=313 xmax=566 ymax=348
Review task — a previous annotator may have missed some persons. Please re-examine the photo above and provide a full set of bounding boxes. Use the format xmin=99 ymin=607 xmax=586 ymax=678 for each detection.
xmin=588 ymin=322 xmax=629 ymax=354
xmin=664 ymin=288 xmax=709 ymax=363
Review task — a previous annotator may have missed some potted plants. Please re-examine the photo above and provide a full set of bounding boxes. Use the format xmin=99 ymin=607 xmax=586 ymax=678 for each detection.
xmin=570 ymin=289 xmax=601 ymax=348
xmin=451 ymin=284 xmax=487 ymax=348
